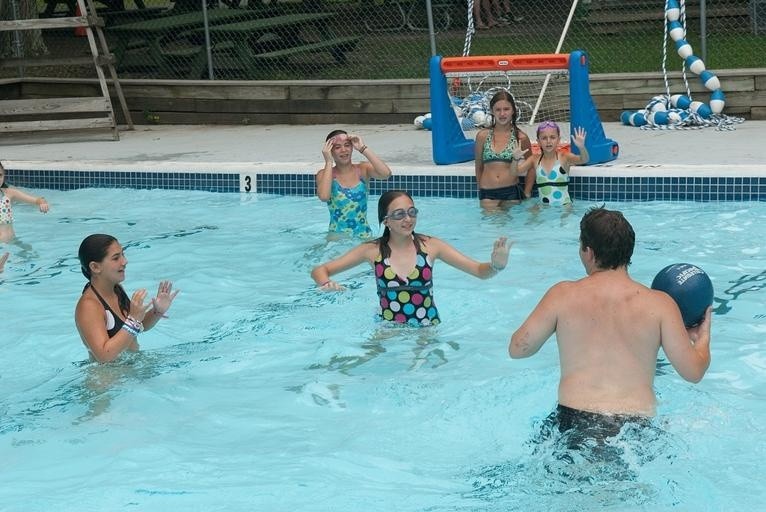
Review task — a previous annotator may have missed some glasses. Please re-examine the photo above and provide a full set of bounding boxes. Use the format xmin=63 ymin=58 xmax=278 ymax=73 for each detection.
xmin=385 ymin=208 xmax=418 ymax=220
xmin=538 ymin=121 xmax=556 ymax=129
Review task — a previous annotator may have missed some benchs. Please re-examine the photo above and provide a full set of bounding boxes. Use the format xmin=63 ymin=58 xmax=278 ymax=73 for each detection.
xmin=86 ymin=0 xmax=365 ymax=81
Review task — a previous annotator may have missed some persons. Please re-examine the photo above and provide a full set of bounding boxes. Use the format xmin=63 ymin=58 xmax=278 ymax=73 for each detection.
xmin=474 ymin=91 xmax=535 ymax=213
xmin=509 ymin=121 xmax=589 ymax=217
xmin=0 ymin=163 xmax=49 ymax=274
xmin=76 ymin=235 xmax=181 ymax=417
xmin=316 ymin=130 xmax=392 ymax=247
xmin=508 ymin=203 xmax=712 ymax=493
xmin=311 ymin=190 xmax=514 ymax=329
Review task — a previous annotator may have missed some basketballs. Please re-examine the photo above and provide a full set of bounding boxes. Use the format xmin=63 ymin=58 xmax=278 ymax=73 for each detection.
xmin=651 ymin=263 xmax=715 ymax=327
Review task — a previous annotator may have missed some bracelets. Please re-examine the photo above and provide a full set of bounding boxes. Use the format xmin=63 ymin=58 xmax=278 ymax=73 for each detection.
xmin=36 ymin=196 xmax=42 ymax=204
xmin=490 ymin=263 xmax=502 ymax=274
xmin=319 ymin=281 xmax=328 ymax=286
xmin=359 ymin=143 xmax=367 ymax=153
xmin=513 ymin=158 xmax=520 ymax=162
xmin=122 ymin=315 xmax=143 ymax=338
xmin=151 ymin=299 xmax=169 ymax=318
